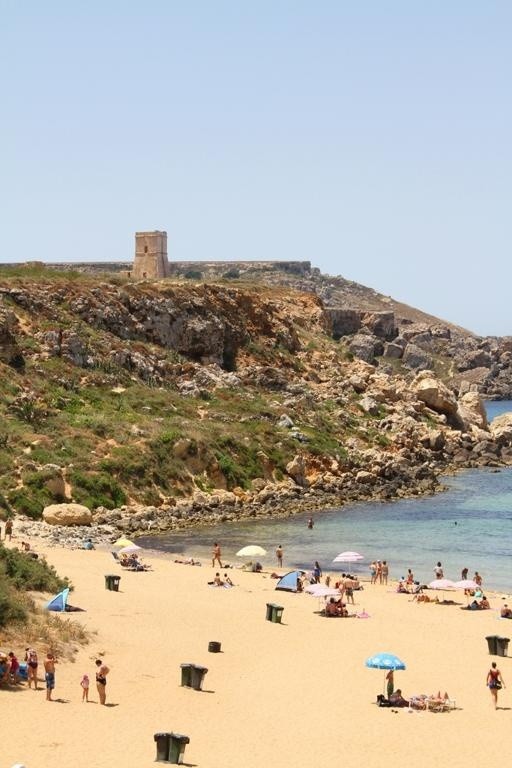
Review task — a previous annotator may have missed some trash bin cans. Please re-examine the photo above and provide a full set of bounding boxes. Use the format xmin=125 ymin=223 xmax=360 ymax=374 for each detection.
xmin=154 ymin=732 xmax=189 ymax=764
xmin=266 ymin=603 xmax=284 ymax=623
xmin=485 ymin=636 xmax=510 ymax=657
xmin=180 ymin=664 xmax=208 ymax=690
xmin=105 ymin=575 xmax=120 ymax=591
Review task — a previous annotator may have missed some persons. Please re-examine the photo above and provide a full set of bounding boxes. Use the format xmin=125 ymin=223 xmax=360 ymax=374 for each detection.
xmin=22 ymin=542 xmax=31 ymax=551
xmin=307 ymin=517 xmax=314 ymax=528
xmin=486 ymin=662 xmax=506 ymax=709
xmin=174 ymin=543 xmax=233 ymax=586
xmin=386 ymin=670 xmax=394 ymax=701
xmin=81 ymin=674 xmax=90 ymax=702
xmin=395 ymin=562 xmax=489 ymax=611
xmin=390 ymin=689 xmax=403 ymax=703
xmin=501 ymin=604 xmax=511 ymax=618
xmin=85 ymin=539 xmax=96 ymax=550
xmin=0 ymin=647 xmax=38 ymax=690
xmin=96 ymin=660 xmax=111 ymax=704
xmin=252 ymin=545 xmax=284 ymax=579
xmin=296 ymin=560 xmax=388 ymax=617
xmin=113 ymin=552 xmax=153 ymax=572
xmin=65 ymin=604 xmax=86 ymax=611
xmin=4 ymin=519 xmax=13 ymax=541
xmin=43 ymin=653 xmax=56 ymax=701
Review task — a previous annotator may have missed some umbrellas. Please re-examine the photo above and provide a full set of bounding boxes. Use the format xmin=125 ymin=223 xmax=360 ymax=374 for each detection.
xmin=365 ymin=652 xmax=406 ymax=696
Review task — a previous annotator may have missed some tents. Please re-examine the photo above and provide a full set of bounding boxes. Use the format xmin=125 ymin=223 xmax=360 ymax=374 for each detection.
xmin=44 ymin=588 xmax=69 ymax=612
xmin=275 ymin=570 xmax=306 ymax=593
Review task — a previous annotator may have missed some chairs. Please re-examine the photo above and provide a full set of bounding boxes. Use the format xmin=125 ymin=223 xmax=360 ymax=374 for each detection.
xmin=108 ymin=550 xmax=155 ymax=572
xmin=405 ymin=694 xmax=458 ymax=713
